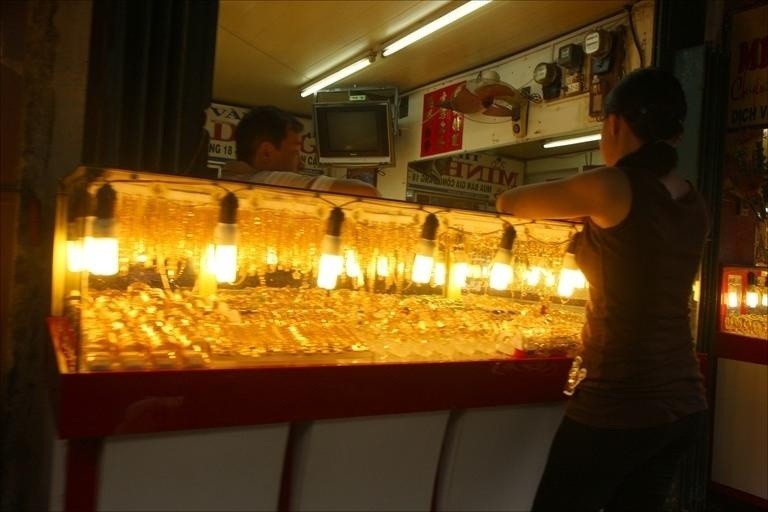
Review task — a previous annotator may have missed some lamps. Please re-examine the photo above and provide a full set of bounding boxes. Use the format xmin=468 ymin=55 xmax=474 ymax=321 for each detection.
xmin=299 ymin=53 xmax=375 ymax=101
xmin=378 ymin=0 xmax=493 ymax=60
xmin=721 ymin=270 xmax=768 ymax=308
xmin=69 ymin=182 xmax=583 ymax=302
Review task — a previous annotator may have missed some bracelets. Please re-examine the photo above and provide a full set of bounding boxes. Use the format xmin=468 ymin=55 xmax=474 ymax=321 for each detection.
xmin=723 ymin=267 xmax=767 ymax=342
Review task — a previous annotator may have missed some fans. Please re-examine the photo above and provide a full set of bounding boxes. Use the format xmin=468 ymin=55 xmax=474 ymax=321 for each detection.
xmin=443 ymin=66 xmax=532 ymax=137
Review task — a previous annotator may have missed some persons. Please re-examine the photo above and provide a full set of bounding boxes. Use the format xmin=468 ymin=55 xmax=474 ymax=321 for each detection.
xmin=205 ymin=103 xmax=384 ymax=202
xmin=492 ymin=62 xmax=721 ymax=510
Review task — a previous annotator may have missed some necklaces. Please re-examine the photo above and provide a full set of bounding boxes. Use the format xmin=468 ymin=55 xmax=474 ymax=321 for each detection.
xmin=61 ymin=192 xmax=591 ymax=377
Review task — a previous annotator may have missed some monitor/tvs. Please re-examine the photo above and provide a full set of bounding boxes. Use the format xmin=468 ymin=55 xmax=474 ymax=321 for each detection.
xmin=313 ymin=103 xmax=393 ymax=163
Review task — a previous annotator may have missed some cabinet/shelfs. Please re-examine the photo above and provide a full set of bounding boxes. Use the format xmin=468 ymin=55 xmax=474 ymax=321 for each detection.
xmin=45 ymin=160 xmax=583 ymax=508
xmin=710 ymin=262 xmax=766 ymax=510
